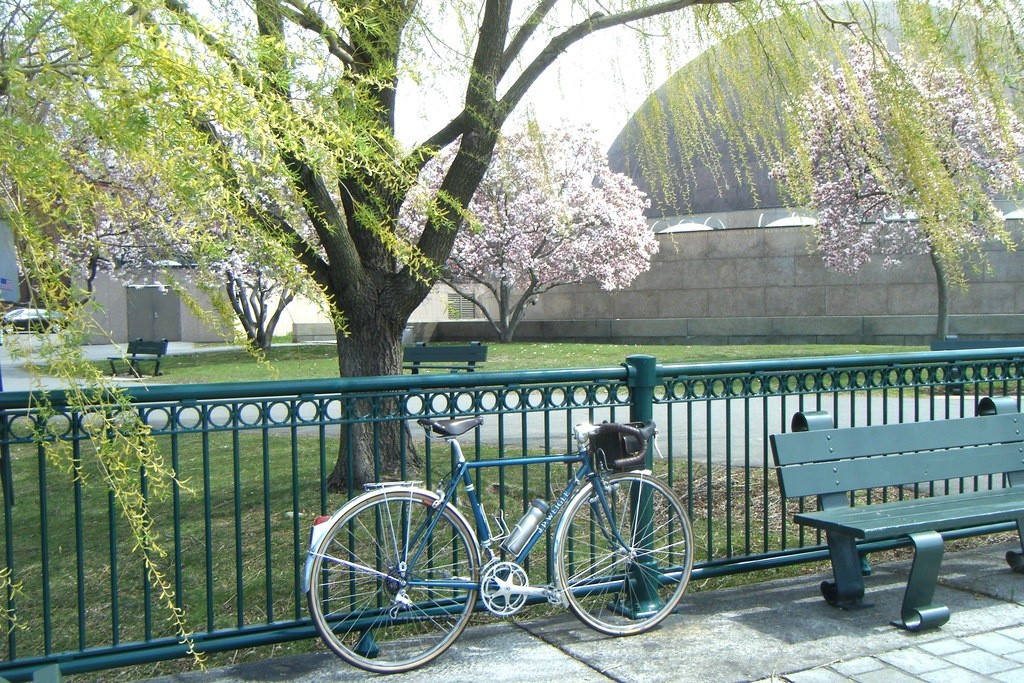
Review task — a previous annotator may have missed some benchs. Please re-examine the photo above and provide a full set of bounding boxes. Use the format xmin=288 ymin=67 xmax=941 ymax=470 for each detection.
xmin=107 ymin=337 xmax=169 ymax=378
xmin=929 ymin=333 xmax=1024 ymax=395
xmin=402 ymin=341 xmax=488 ymax=375
xmin=769 ymin=395 xmax=1024 ymax=633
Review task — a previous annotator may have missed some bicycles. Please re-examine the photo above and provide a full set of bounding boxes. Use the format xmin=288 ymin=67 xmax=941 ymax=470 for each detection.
xmin=302 ymin=415 xmax=696 ymax=676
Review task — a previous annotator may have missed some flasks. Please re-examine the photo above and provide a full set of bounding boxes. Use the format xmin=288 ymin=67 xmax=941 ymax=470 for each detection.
xmin=500 ymin=493 xmax=553 ymax=557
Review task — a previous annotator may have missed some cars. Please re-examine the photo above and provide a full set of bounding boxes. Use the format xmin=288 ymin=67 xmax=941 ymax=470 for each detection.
xmin=0 ymin=306 xmax=72 ymax=334
